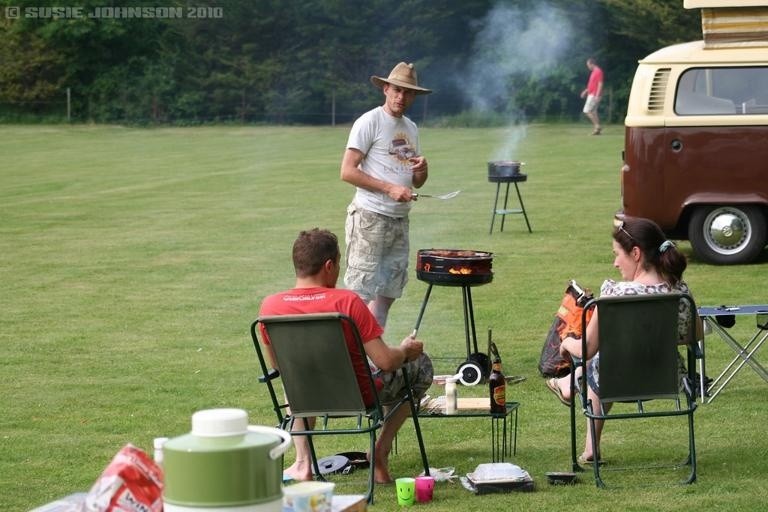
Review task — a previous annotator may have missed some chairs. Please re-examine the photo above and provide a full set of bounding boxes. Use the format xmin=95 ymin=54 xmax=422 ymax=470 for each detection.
xmin=250 ymin=311 xmax=432 ymax=506
xmin=568 ymin=293 xmax=699 ymax=490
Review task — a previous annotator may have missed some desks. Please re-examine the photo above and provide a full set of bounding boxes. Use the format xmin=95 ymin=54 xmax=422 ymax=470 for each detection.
xmin=690 ymin=304 xmax=767 ymax=406
xmin=364 ymin=399 xmax=521 ymax=466
xmin=24 ymin=488 xmax=373 ymax=512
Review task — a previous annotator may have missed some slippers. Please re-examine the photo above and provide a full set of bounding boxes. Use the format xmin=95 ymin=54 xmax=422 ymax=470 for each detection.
xmin=578 ymin=453 xmax=606 ymax=465
xmin=546 ymin=378 xmax=571 ymax=406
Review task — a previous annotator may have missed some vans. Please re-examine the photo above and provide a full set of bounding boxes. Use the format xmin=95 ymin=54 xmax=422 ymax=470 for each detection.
xmin=619 ymin=1 xmax=767 ymax=266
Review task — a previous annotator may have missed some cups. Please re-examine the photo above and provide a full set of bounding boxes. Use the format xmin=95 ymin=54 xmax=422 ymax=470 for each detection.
xmin=413 ymin=474 xmax=436 ymax=502
xmin=396 ymin=476 xmax=417 ymax=508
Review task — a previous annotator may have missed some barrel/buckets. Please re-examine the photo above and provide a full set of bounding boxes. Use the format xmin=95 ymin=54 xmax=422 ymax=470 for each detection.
xmin=161 ymin=406 xmax=293 ymax=512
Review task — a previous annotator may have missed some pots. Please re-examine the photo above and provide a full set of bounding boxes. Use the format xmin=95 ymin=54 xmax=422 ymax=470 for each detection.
xmin=486 ymin=161 xmax=521 ymax=181
xmin=418 ymin=248 xmax=493 ymax=274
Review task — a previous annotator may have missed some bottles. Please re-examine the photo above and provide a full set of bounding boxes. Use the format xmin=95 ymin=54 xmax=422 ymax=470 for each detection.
xmin=488 ymin=356 xmax=508 ymax=418
xmin=443 ymin=376 xmax=459 ymax=416
xmin=152 ymin=437 xmax=171 ymax=468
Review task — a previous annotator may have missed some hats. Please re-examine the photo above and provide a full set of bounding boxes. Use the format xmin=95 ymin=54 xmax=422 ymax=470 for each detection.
xmin=371 ymin=63 xmax=434 ymax=94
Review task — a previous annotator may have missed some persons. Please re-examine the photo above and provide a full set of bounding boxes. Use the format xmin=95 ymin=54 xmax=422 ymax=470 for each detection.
xmin=579 ymin=57 xmax=604 ymax=136
xmin=337 ymin=62 xmax=433 ymax=340
xmin=257 ymin=225 xmax=434 ymax=485
xmin=545 ymin=210 xmax=689 ymax=465
